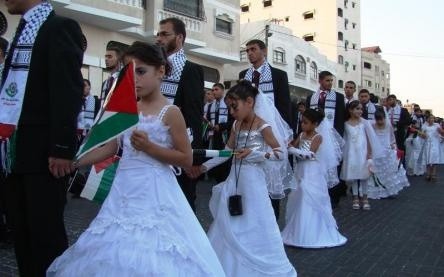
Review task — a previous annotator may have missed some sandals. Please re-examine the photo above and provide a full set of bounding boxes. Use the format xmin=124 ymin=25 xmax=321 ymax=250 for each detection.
xmin=352 ymin=198 xmax=360 ymax=209
xmin=362 ymin=199 xmax=371 ymax=210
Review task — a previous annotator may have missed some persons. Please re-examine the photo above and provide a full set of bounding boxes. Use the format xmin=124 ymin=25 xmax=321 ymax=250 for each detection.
xmin=201 ymin=83 xmax=237 ymax=185
xmin=66 ymin=79 xmax=100 ymax=178
xmin=153 ymin=17 xmax=205 ymax=212
xmin=98 ymin=40 xmax=130 ymax=109
xmin=306 ymin=72 xmax=347 ymax=208
xmin=1 ymin=0 xmax=86 ymax=277
xmin=358 ymin=87 xmax=444 ymax=199
xmin=342 ymin=80 xmax=357 ymax=120
xmin=44 ymin=40 xmax=229 ymax=276
xmin=186 ymin=78 xmax=299 ymax=277
xmin=296 ymin=101 xmax=305 ymax=136
xmin=280 ymin=107 xmax=349 ymax=250
xmin=338 ymin=100 xmax=376 ymax=210
xmin=238 ymin=40 xmax=293 ymax=221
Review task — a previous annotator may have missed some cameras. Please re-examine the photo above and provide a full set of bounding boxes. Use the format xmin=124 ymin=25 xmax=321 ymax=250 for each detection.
xmin=228 ymin=195 xmax=243 ymax=216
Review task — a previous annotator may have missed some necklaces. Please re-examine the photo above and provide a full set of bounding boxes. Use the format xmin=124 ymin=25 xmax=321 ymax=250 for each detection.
xmin=303 ymin=131 xmax=315 ymax=140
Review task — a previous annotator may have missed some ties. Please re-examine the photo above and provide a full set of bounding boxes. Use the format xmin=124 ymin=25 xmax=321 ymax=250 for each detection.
xmin=319 ymin=92 xmax=327 ymax=109
xmin=252 ymin=70 xmax=260 ymax=88
xmin=109 ymin=77 xmax=114 ymax=91
xmin=1 ymin=17 xmax=27 ymax=92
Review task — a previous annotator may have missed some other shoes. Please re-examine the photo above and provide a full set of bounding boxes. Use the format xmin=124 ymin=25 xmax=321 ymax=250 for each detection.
xmin=431 ymin=175 xmax=437 ymax=182
xmin=425 ymin=175 xmax=430 ymax=181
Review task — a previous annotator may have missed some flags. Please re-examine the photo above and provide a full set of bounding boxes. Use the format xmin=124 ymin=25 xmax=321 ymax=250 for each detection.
xmin=67 ymin=153 xmax=120 ymax=203
xmin=73 ymin=59 xmax=140 ymax=161
